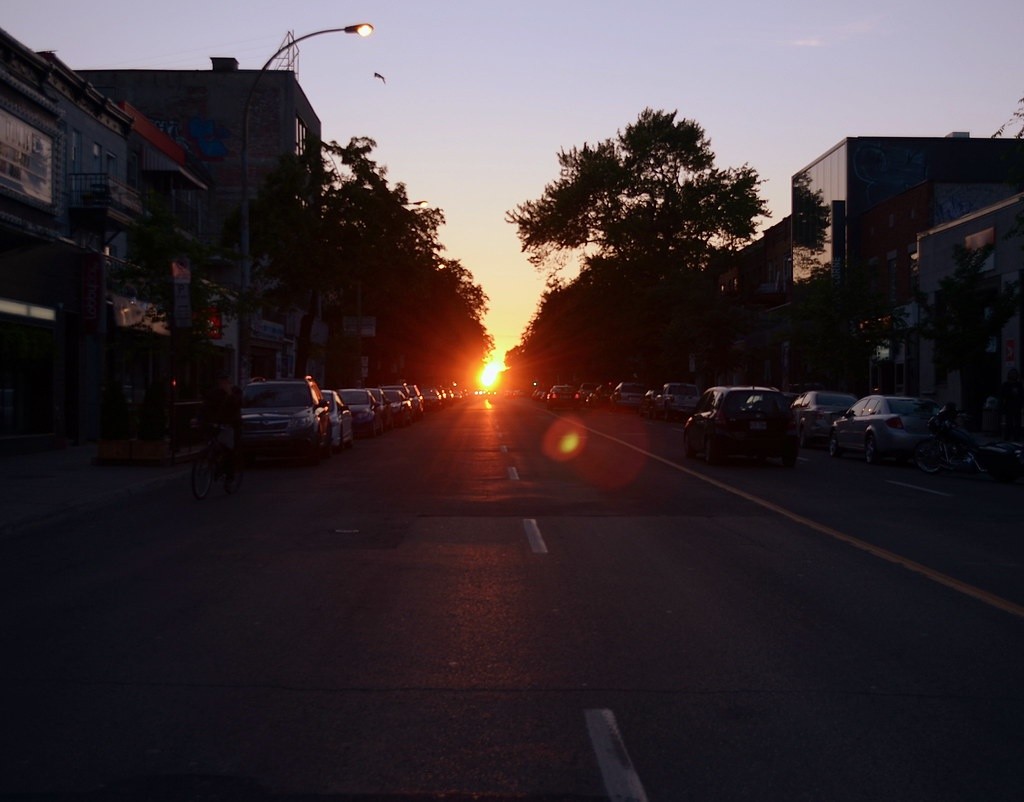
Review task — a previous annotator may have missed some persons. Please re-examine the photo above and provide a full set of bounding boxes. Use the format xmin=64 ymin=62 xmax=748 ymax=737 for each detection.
xmin=189 ymin=370 xmax=243 ymax=482
xmin=1000 ymin=368 xmax=1024 ymax=442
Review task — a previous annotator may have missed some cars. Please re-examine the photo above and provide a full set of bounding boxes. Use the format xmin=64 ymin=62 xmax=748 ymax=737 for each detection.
xmin=683 ymin=387 xmax=800 ymax=467
xmin=780 ymin=391 xmax=943 ymax=465
xmin=321 ymin=380 xmax=462 ymax=457
xmin=532 ymin=382 xmax=698 ymax=422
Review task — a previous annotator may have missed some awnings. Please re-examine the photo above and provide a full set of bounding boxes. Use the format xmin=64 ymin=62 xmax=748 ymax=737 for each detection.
xmin=141 ymin=145 xmax=208 ymax=192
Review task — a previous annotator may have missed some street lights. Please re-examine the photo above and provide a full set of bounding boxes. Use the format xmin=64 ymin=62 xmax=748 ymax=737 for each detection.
xmin=239 ymin=25 xmax=374 ymax=390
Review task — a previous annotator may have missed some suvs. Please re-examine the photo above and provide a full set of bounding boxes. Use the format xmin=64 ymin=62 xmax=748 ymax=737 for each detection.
xmin=240 ymin=376 xmax=329 ymax=465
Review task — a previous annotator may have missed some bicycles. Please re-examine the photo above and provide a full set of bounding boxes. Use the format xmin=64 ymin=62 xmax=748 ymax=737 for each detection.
xmin=187 ymin=420 xmax=248 ymax=501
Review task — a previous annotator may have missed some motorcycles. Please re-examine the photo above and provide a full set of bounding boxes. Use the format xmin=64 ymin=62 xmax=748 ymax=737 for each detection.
xmin=912 ymin=401 xmax=1024 ymax=483
